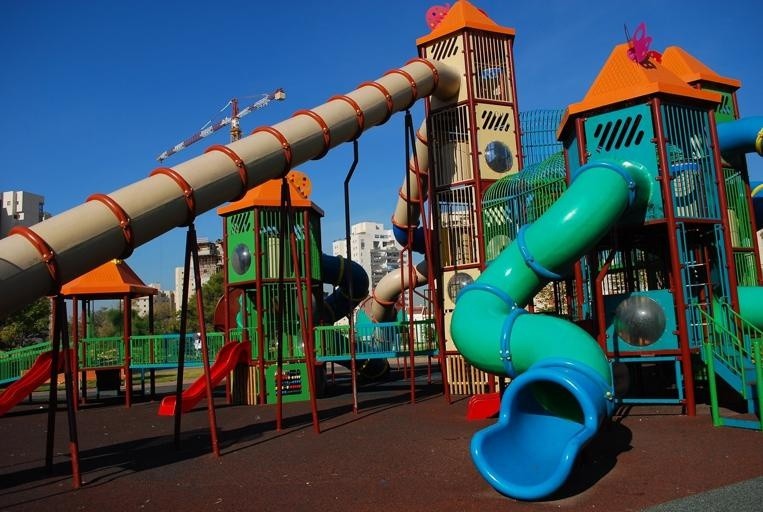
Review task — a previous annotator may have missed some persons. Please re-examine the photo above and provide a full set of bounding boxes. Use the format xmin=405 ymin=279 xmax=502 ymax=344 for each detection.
xmin=193 ymin=332 xmax=203 ymax=360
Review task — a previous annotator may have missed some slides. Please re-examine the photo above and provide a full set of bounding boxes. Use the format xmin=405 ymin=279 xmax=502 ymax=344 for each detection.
xmin=0 ymin=348 xmax=73 ymax=418
xmin=158 ymin=342 xmax=243 ymax=417
